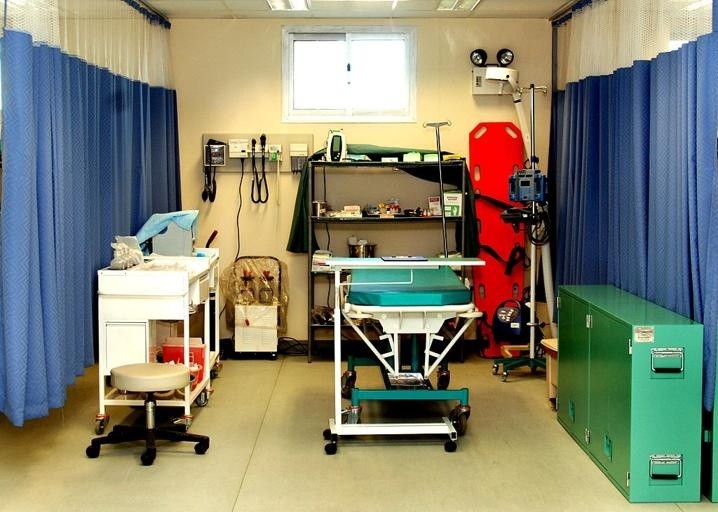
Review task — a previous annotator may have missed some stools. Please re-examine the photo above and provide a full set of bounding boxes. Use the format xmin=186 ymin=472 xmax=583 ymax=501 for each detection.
xmin=86 ymin=364 xmax=210 ymax=465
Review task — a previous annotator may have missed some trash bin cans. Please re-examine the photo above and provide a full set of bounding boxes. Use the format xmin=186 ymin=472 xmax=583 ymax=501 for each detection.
xmin=538 ymin=338 xmax=559 ymax=410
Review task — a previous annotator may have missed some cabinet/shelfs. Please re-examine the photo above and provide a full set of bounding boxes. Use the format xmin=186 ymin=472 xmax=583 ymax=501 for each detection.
xmin=314 ymin=256 xmax=485 ymax=454
xmin=306 ymin=160 xmax=468 ymax=364
xmin=94 ymin=247 xmax=222 ymax=434
xmin=557 ymin=284 xmax=704 ymax=504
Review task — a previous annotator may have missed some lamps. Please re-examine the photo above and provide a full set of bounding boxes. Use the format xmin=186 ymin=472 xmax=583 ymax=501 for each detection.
xmin=455 ymin=0 xmax=483 ymax=17
xmin=437 ymin=0 xmax=459 ymax=12
xmin=470 ymin=48 xmax=519 ymax=95
